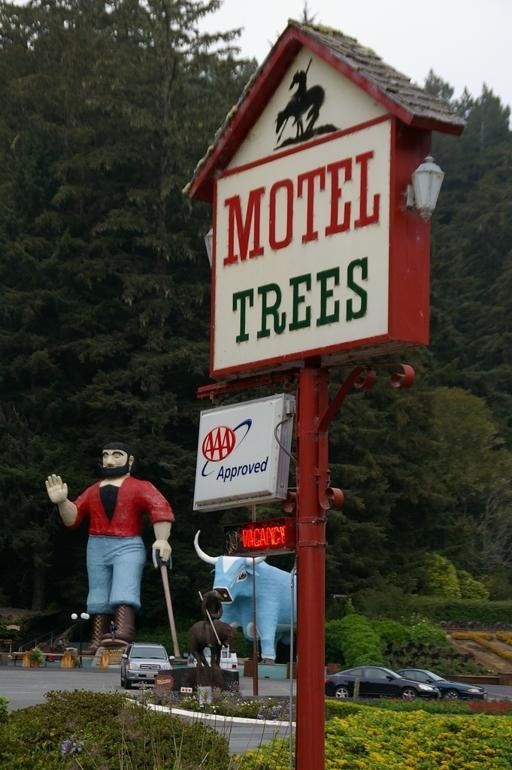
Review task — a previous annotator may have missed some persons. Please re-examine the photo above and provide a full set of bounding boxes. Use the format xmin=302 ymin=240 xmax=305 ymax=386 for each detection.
xmin=56 ymin=638 xmax=66 ymax=650
xmin=44 ymin=440 xmax=176 ymax=655
xmin=109 ymin=620 xmax=120 ymax=641
xmin=200 ymin=590 xmax=225 ymax=621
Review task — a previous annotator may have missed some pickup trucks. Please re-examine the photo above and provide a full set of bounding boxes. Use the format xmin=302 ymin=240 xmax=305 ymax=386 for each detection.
xmin=187 ymin=642 xmax=239 ymax=673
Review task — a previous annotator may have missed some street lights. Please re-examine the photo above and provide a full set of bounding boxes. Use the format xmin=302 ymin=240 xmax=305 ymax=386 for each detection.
xmin=71 ymin=612 xmax=90 ymax=667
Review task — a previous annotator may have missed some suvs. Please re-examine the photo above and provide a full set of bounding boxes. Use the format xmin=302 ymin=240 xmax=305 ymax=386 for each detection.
xmin=120 ymin=642 xmax=175 ymax=690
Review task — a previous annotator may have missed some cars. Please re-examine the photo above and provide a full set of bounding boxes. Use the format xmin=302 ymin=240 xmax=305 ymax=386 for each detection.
xmin=395 ymin=668 xmax=489 ymax=704
xmin=325 ymin=666 xmax=442 ymax=703
